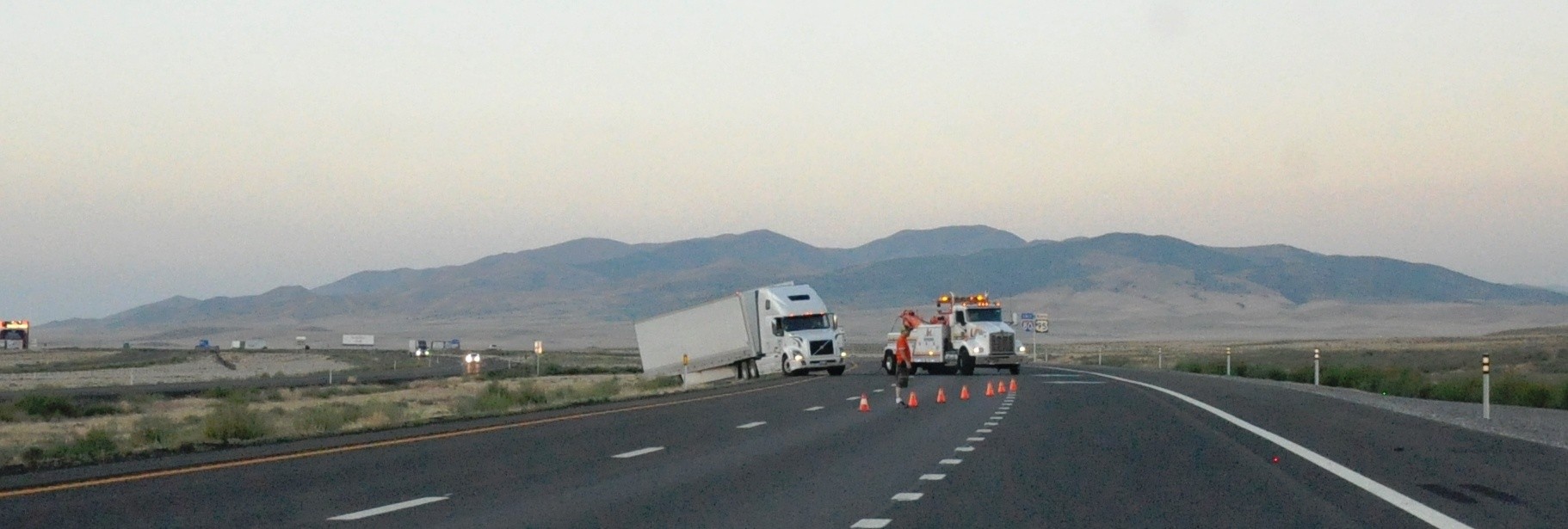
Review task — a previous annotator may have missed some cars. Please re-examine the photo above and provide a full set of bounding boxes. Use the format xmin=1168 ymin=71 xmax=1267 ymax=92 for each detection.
xmin=466 ymin=352 xmax=481 ymax=363
xmin=416 ymin=348 xmax=429 ymax=358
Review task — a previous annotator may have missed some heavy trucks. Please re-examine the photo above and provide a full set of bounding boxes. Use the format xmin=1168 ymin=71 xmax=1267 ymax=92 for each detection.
xmin=634 ymin=280 xmax=847 ymax=382
xmin=882 ymin=291 xmax=1026 ymax=377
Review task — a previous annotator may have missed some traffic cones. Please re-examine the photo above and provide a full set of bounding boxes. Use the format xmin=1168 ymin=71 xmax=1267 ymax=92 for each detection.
xmin=857 ymin=392 xmax=872 ymax=412
xmin=959 ymin=384 xmax=971 ymax=400
xmin=907 ymin=391 xmax=919 ymax=408
xmin=1010 ymin=378 xmax=1017 ymax=392
xmin=985 ymin=381 xmax=995 ymax=397
xmin=997 ymin=380 xmax=1007 ymax=393
xmin=935 ymin=387 xmax=947 ymax=403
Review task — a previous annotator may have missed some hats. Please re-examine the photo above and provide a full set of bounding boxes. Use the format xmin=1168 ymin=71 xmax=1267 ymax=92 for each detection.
xmin=901 ymin=325 xmax=913 ymax=331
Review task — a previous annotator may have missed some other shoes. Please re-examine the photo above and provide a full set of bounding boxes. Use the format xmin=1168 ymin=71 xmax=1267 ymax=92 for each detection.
xmin=897 ymin=400 xmax=908 ymax=408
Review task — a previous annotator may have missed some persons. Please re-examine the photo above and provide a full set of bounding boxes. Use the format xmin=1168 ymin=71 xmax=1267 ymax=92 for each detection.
xmin=896 ymin=326 xmax=911 ymax=403
